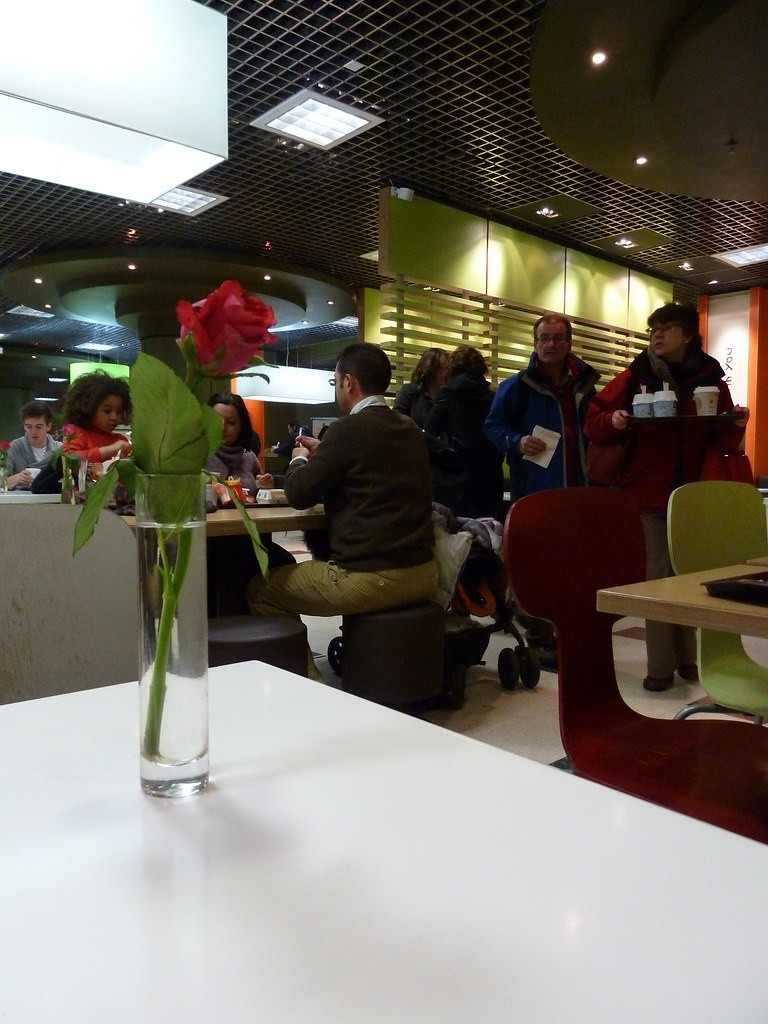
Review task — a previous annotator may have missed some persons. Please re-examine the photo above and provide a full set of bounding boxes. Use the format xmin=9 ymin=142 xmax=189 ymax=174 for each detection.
xmin=391 ymin=345 xmax=507 ymax=633
xmin=0 ymin=401 xmax=62 ymax=490
xmin=584 ymin=301 xmax=749 ymax=692
xmin=201 ymin=392 xmax=297 ymax=619
xmin=483 ymin=314 xmax=601 ymax=674
xmin=60 ymin=371 xmax=134 ymax=479
xmin=246 ymin=343 xmax=438 ymax=686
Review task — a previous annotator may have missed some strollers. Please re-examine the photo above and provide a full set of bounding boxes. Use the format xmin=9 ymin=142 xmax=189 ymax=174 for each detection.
xmin=327 ymin=503 xmax=542 ymax=691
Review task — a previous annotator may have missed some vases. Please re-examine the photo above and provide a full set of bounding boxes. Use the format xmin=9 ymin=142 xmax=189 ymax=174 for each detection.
xmin=134 ymin=472 xmax=211 ymax=798
xmin=60 ymin=469 xmax=75 ymax=505
xmin=0 ymin=467 xmax=8 ymax=493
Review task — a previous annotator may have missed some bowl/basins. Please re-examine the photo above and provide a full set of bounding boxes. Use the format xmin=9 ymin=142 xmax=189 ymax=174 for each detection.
xmin=256 ymin=489 xmax=287 ymax=504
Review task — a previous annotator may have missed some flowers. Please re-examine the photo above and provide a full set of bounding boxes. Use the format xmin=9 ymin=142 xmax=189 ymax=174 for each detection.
xmin=70 ymin=278 xmax=278 ymax=754
xmin=0 ymin=441 xmax=11 ymax=490
xmin=49 ymin=424 xmax=83 ymax=502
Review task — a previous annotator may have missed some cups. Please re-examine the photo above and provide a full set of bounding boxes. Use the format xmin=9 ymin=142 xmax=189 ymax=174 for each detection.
xmin=25 ymin=468 xmax=41 ymax=486
xmin=653 ymin=391 xmax=678 ymax=417
xmin=693 ymin=386 xmax=720 ymax=415
xmin=632 ymin=394 xmax=654 ymax=417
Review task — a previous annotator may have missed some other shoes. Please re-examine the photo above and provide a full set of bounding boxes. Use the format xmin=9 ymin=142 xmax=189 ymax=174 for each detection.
xmin=677 ymin=660 xmax=698 ymax=682
xmin=529 ymin=642 xmax=559 ymax=673
xmin=643 ymin=674 xmax=671 ymax=692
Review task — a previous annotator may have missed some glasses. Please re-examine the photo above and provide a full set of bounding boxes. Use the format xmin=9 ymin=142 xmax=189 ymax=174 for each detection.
xmin=646 ymin=325 xmax=686 ymax=334
xmin=329 ymin=375 xmax=345 ymax=385
xmin=535 ymin=335 xmax=568 ymax=343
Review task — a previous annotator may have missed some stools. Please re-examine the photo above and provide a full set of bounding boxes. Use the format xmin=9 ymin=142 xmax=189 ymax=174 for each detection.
xmin=341 ymin=599 xmax=444 ymax=722
xmin=208 ymin=616 xmax=308 ymax=678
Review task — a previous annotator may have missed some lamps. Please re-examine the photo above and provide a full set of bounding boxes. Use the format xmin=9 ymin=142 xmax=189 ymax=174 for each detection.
xmin=70 ymin=349 xmax=130 ymax=385
xmin=230 ymin=333 xmax=335 ymax=404
xmin=0 ymin=0 xmax=228 ymax=206
xmin=391 ymin=186 xmax=415 ymax=203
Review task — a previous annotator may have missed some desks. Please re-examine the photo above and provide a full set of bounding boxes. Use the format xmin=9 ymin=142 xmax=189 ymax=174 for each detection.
xmin=0 ymin=660 xmax=768 ymax=1024
xmin=257 ymin=450 xmax=285 ymax=458
xmin=596 ymin=564 xmax=768 ymax=639
xmin=118 ymin=504 xmax=328 ymax=622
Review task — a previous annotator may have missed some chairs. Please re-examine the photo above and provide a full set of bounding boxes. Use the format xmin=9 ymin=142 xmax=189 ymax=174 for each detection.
xmin=667 ymin=480 xmax=768 ymax=726
xmin=0 ymin=503 xmax=141 ymax=705
xmin=503 ymin=488 xmax=768 ymax=846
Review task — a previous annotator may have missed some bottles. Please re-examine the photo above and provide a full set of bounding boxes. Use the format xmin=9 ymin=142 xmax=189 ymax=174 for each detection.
xmin=201 ymin=469 xmax=255 ymax=513
xmin=61 ymin=469 xmax=75 ymax=505
xmin=0 ymin=454 xmax=8 ymax=495
